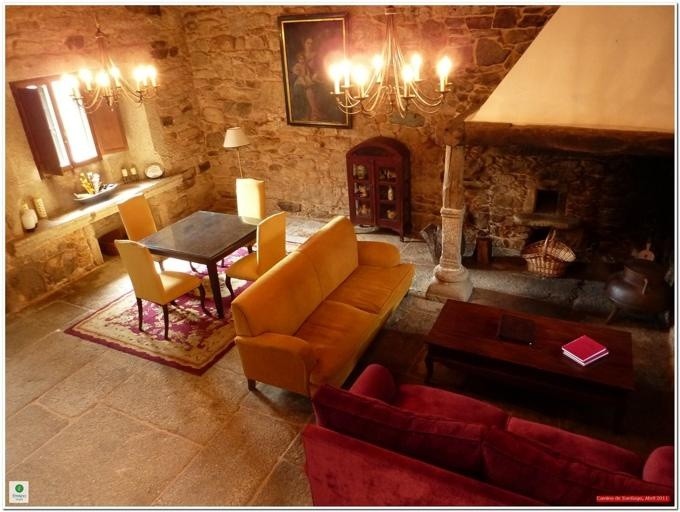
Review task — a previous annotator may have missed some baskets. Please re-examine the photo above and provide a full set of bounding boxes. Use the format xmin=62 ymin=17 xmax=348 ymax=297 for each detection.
xmin=521 ymin=228 xmax=576 ymax=278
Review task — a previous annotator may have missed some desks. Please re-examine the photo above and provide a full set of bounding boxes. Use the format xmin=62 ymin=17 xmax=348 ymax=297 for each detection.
xmin=6 ymin=172 xmax=184 ymax=258
xmin=425 ymin=299 xmax=632 ymax=435
xmin=139 ymin=210 xmax=263 ymax=318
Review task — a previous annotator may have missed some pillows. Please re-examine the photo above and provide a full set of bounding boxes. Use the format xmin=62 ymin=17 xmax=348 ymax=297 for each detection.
xmin=306 ymin=374 xmax=483 ymax=477
xmin=485 ymin=422 xmax=673 ymax=504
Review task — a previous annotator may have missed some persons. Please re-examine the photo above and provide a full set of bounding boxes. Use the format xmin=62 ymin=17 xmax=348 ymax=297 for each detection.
xmin=292 ymin=37 xmax=321 ymax=122
xmin=292 ymin=52 xmax=316 ymax=90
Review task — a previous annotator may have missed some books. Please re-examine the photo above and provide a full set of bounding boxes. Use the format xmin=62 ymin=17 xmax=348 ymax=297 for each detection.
xmin=563 ymin=349 xmax=610 ymax=367
xmin=498 ymin=314 xmax=537 ymax=345
xmin=561 ymin=334 xmax=606 ymax=362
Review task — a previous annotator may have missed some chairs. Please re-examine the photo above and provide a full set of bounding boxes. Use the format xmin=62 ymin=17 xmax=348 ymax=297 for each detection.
xmin=117 ymin=195 xmax=173 ymax=269
xmin=229 ymin=177 xmax=267 ymax=254
xmin=225 ymin=210 xmax=289 ymax=301
xmin=109 ymin=238 xmax=212 ymax=341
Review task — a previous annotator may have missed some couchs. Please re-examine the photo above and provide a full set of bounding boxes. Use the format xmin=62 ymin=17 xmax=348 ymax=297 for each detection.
xmin=230 ymin=216 xmax=415 ymax=400
xmin=304 ymin=358 xmax=674 ymax=505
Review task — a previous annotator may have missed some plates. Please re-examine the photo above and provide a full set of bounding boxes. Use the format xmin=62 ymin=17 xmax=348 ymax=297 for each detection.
xmin=144 ymin=162 xmax=164 ymax=179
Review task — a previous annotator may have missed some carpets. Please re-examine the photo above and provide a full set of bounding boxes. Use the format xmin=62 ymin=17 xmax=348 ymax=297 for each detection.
xmin=63 ymin=240 xmax=303 ymax=377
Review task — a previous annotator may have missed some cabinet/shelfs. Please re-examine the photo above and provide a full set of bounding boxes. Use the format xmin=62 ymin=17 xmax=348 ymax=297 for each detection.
xmin=345 ymin=134 xmax=411 ymax=240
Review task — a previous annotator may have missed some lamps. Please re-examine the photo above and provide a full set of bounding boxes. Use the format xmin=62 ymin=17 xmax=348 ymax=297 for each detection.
xmin=327 ymin=6 xmax=456 ymax=119
xmin=62 ymin=6 xmax=163 ymax=114
xmin=223 ymin=125 xmax=250 ymax=179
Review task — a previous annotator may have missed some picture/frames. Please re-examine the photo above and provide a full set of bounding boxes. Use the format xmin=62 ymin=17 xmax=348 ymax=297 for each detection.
xmin=277 ymin=12 xmax=352 ymax=129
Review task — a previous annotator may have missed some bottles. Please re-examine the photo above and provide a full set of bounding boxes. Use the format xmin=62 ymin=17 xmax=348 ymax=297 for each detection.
xmin=21 ymin=204 xmax=35 ymax=232
xmin=33 ymin=197 xmax=48 ymax=219
